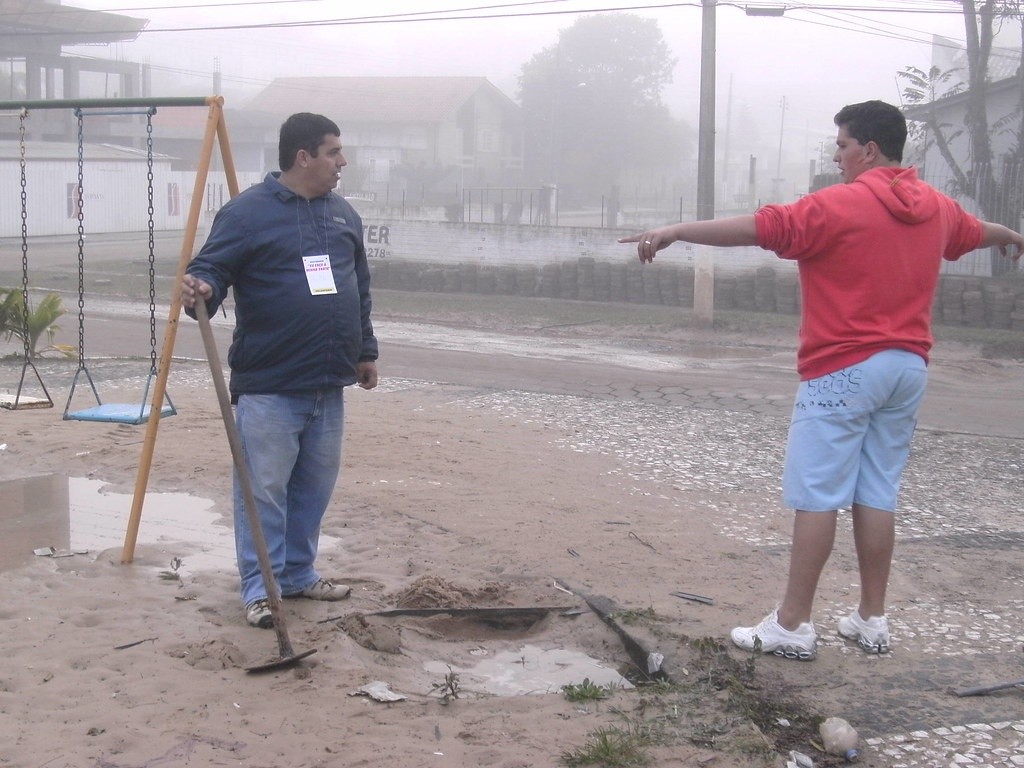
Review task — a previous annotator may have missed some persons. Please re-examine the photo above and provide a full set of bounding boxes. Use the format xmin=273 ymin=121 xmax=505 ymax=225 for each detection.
xmin=618 ymin=94 xmax=1024 ymax=660
xmin=182 ymin=110 xmax=380 ymax=631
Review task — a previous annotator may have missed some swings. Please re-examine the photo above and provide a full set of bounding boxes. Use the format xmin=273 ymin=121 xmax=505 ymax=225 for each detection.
xmin=62 ymin=106 xmax=178 ymax=426
xmin=0 ymin=109 xmax=54 ymax=412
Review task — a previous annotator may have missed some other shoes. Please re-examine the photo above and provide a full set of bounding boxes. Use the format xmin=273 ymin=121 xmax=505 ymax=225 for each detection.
xmin=247 ymin=597 xmax=282 ymax=628
xmin=302 ymin=577 xmax=351 ymax=601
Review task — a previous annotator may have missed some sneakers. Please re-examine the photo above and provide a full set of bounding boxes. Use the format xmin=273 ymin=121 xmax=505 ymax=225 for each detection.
xmin=838 ymin=608 xmax=892 ymax=654
xmin=730 ymin=607 xmax=820 ymax=661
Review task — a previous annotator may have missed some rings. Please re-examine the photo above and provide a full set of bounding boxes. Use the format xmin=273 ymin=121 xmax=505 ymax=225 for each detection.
xmin=644 ymin=240 xmax=651 ymax=245
